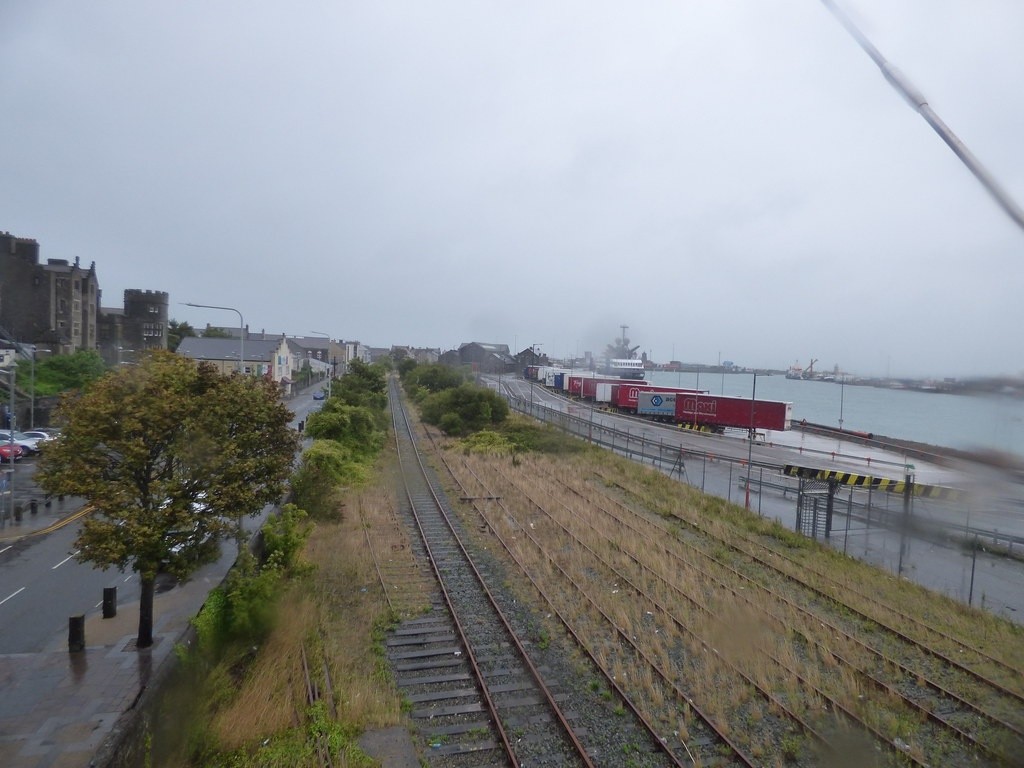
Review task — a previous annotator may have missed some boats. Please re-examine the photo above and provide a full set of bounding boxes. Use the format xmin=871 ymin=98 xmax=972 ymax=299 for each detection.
xmin=784 ymin=359 xmax=820 ymax=381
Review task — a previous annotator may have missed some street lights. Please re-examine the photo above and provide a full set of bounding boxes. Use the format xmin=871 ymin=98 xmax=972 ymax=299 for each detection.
xmin=118 ymin=347 xmax=135 ymax=365
xmin=180 ymin=301 xmax=246 ymax=375
xmin=529 ymin=342 xmax=543 ymax=418
xmin=311 ymin=331 xmax=331 ymax=399
xmin=31 ymin=347 xmax=51 ymax=430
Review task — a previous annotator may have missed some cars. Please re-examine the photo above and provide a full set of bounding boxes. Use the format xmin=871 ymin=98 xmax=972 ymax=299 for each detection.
xmin=22 ymin=427 xmax=66 ymax=443
xmin=312 ymin=389 xmax=325 ymax=401
xmin=0 ymin=439 xmax=23 ymax=463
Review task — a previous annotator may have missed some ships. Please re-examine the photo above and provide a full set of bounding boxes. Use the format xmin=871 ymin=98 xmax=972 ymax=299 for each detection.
xmin=594 ymin=322 xmax=646 ymax=380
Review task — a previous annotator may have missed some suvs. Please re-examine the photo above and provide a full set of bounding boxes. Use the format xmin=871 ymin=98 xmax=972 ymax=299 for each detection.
xmin=0 ymin=429 xmax=39 ymax=457
